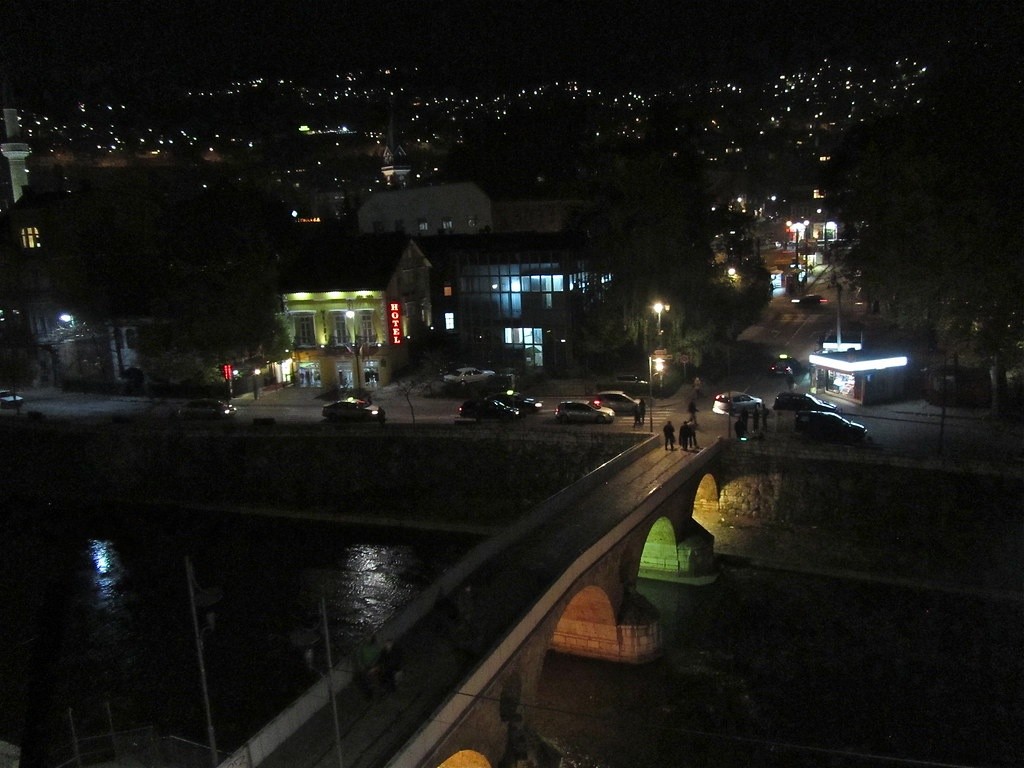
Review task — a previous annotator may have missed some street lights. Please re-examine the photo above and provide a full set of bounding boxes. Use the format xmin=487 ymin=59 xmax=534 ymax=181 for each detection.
xmin=654 ymin=302 xmax=664 ymax=399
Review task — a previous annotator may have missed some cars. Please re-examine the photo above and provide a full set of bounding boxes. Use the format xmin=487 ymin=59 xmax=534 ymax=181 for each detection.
xmin=773 ymin=391 xmax=837 ymax=412
xmin=795 ymin=293 xmax=823 ymax=305
xmin=794 ymin=410 xmax=867 ymax=444
xmin=320 ymin=396 xmax=386 ymax=424
xmin=554 ymin=399 xmax=615 ymax=425
xmin=439 ymin=361 xmax=544 ymax=424
xmin=0 ymin=389 xmax=24 ymax=409
xmin=767 ymin=354 xmax=802 ymax=378
xmin=597 ymin=390 xmax=642 ymax=415
xmin=712 ymin=390 xmax=763 ymax=417
xmin=616 ymin=374 xmax=649 ymax=387
xmin=175 ymin=397 xmax=238 ymax=420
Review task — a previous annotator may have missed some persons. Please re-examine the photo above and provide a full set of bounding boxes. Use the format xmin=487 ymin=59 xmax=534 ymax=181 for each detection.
xmin=735 ymin=403 xmax=768 ymax=439
xmin=375 ymin=641 xmax=406 ymax=694
xmin=631 ymin=403 xmax=641 ymax=427
xmin=689 ymin=398 xmax=700 ymax=425
xmin=687 ymin=419 xmax=697 ymax=447
xmin=681 ymin=420 xmax=690 ymax=451
xmin=639 ymin=398 xmax=646 ymax=424
xmin=663 ymin=421 xmax=675 ymax=451
